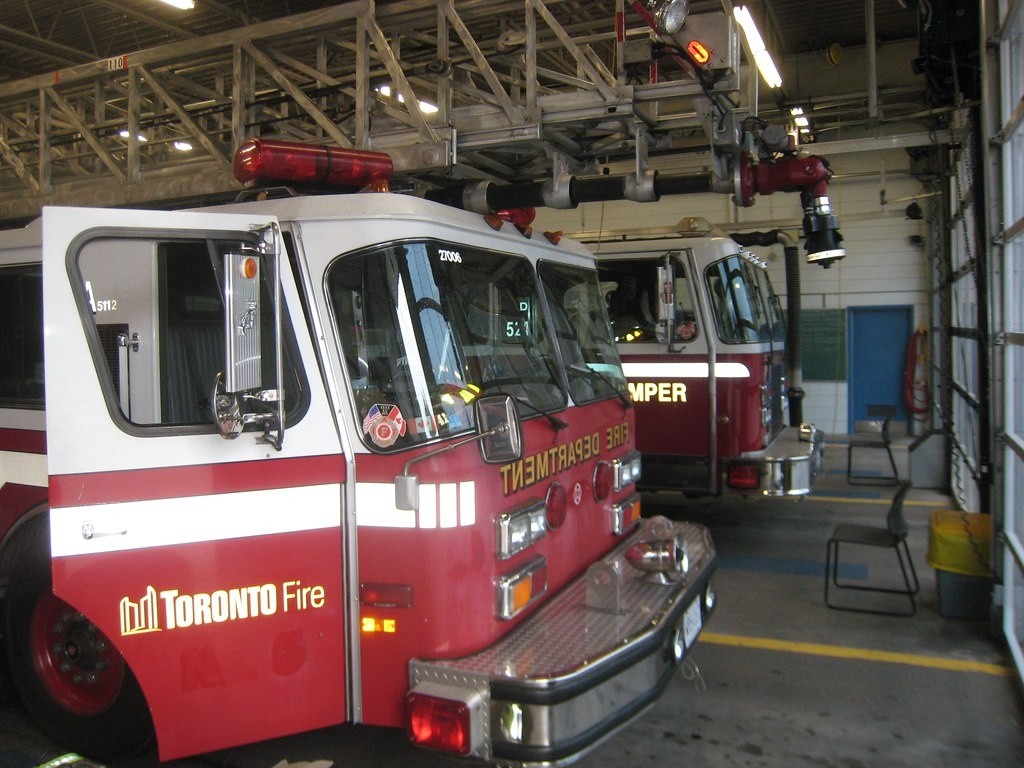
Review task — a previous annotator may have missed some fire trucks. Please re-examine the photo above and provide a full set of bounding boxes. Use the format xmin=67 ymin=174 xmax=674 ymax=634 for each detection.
xmin=1 ymin=141 xmax=718 ymax=768
xmin=458 ymin=213 xmax=825 ymax=512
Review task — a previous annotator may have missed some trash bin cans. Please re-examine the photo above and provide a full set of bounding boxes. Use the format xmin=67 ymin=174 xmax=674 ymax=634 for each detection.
xmin=908 ymin=429 xmax=948 ymax=490
xmin=927 ymin=509 xmax=991 ymax=621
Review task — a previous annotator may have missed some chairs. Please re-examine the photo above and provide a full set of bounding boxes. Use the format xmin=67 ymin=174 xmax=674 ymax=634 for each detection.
xmin=824 ymin=481 xmax=919 ymax=618
xmin=846 ymin=404 xmax=900 ymax=487
